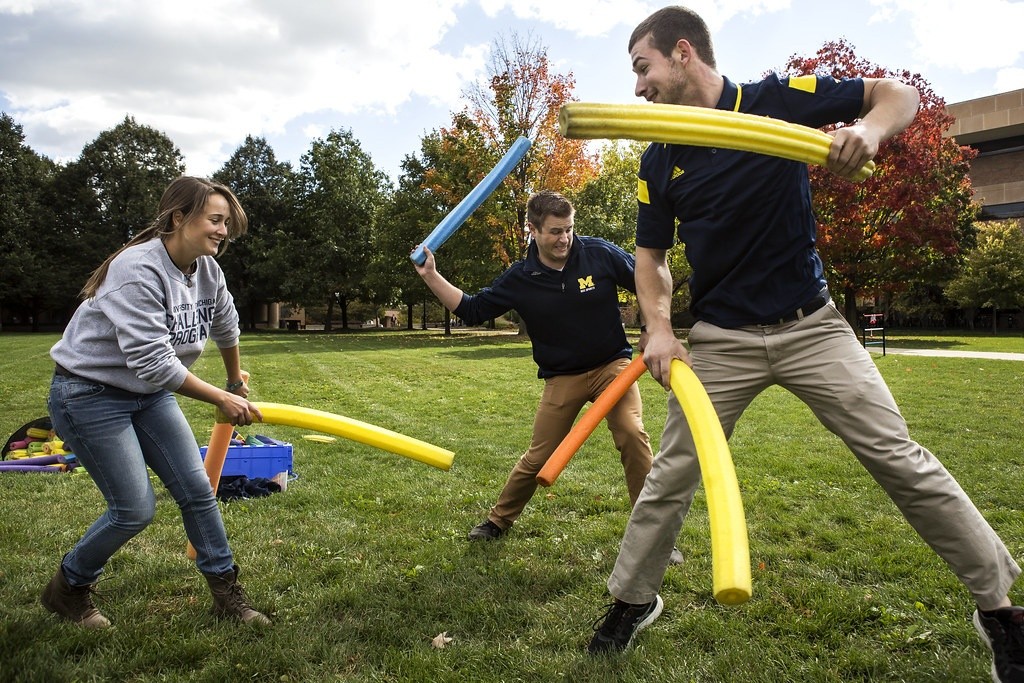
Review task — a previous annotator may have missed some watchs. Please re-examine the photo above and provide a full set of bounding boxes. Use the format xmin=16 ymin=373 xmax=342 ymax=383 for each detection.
xmin=226 ymin=378 xmax=244 ymax=390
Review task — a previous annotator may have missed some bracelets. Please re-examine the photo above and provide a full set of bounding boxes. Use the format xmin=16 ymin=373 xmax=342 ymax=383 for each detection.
xmin=640 ymin=325 xmax=646 ymax=333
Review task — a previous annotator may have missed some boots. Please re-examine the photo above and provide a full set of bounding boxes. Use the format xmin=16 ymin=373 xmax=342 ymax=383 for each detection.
xmin=203 ymin=566 xmax=272 ymax=628
xmin=40 ymin=552 xmax=112 ymax=630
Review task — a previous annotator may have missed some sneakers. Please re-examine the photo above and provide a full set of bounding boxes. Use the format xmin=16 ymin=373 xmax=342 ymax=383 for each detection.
xmin=668 ymin=546 xmax=684 ymax=565
xmin=467 ymin=519 xmax=503 ymax=541
xmin=588 ymin=595 xmax=664 ymax=656
xmin=972 ymin=601 xmax=1024 ymax=683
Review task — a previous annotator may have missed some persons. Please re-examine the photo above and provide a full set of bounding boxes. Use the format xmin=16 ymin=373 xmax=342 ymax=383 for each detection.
xmin=41 ymin=176 xmax=274 ymax=630
xmin=585 ymin=5 xmax=1024 ymax=683
xmin=410 ymin=192 xmax=684 ymax=563
xmin=391 ymin=314 xmax=397 ymax=326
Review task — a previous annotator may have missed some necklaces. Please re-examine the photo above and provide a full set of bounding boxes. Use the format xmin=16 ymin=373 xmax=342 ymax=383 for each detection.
xmin=161 ymin=238 xmax=195 ymax=287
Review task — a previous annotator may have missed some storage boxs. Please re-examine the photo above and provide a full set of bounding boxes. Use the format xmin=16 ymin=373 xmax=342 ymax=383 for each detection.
xmin=200 ymin=436 xmax=293 ymax=480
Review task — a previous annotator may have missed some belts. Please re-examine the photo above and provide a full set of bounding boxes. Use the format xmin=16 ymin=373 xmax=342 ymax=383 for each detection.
xmin=746 ymin=285 xmax=831 ymax=328
